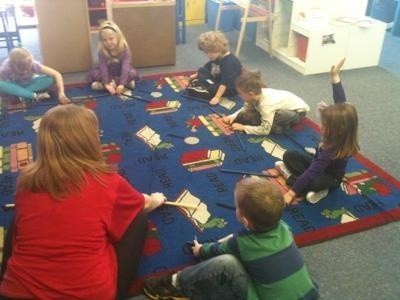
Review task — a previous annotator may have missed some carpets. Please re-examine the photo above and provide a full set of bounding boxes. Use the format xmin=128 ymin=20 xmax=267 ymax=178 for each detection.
xmin=0 ymin=68 xmax=400 ymax=300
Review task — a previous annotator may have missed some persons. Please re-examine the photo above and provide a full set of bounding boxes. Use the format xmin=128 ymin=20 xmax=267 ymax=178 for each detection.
xmin=190 ymin=29 xmax=244 ymax=106
xmin=1 ymin=102 xmax=169 ymax=300
xmin=141 ymin=177 xmax=323 ymax=300
xmin=0 ymin=46 xmax=70 ymax=105
xmin=221 ymin=69 xmax=311 ymax=136
xmin=87 ymin=20 xmax=141 ymax=96
xmin=273 ymin=55 xmax=361 ymax=209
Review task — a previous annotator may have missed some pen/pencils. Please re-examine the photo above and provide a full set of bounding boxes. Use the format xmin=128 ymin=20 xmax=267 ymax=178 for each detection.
xmin=216 ymin=202 xmax=236 ymax=210
xmin=163 ymin=202 xmax=198 ymax=209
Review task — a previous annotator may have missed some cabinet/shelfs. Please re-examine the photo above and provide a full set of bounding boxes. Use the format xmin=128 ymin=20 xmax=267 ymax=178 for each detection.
xmin=86 ymin=0 xmax=113 ymax=65
xmin=256 ymin=0 xmax=387 ymax=76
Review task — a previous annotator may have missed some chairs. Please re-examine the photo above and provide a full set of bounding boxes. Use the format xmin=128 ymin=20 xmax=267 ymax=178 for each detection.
xmin=0 ymin=7 xmax=21 ymax=47
xmin=0 ymin=11 xmax=14 ymax=52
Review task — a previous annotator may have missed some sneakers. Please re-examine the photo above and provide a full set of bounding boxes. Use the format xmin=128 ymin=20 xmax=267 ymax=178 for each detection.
xmin=91 ymin=80 xmax=117 ymax=91
xmin=186 ymin=79 xmax=210 ymax=98
xmin=306 ymin=189 xmax=329 ymax=205
xmin=274 ymin=161 xmax=291 ymax=180
xmin=128 ymin=80 xmax=135 ymax=89
xmin=33 ymin=92 xmax=50 ymax=101
xmin=142 ymin=272 xmax=192 ymax=300
